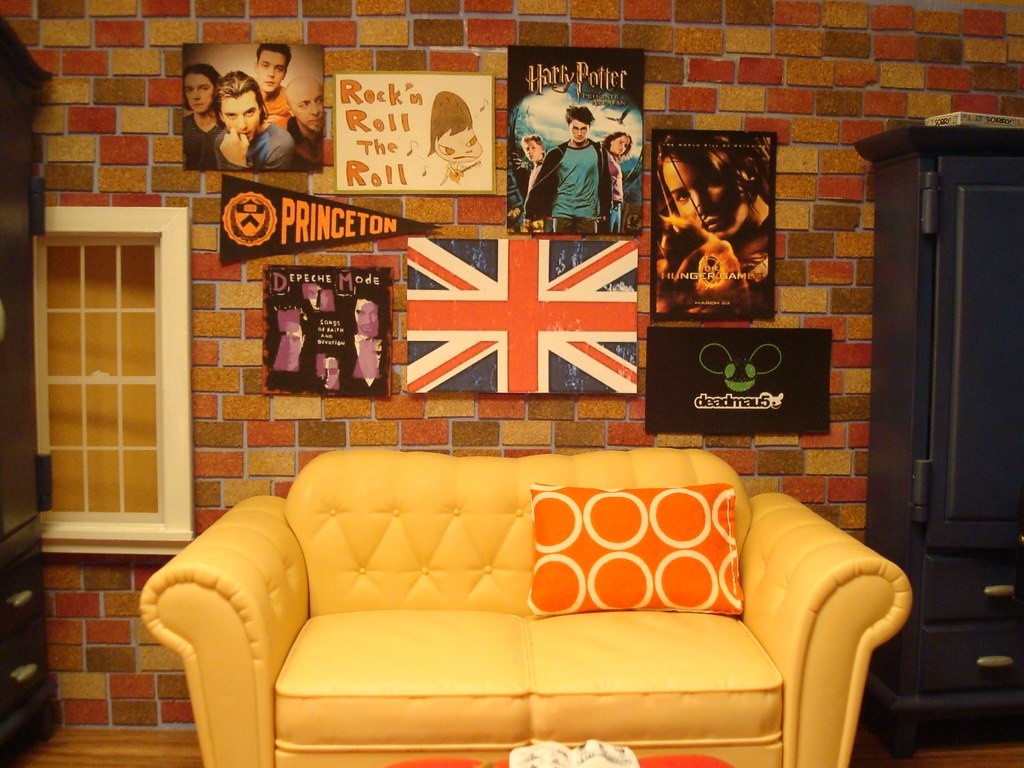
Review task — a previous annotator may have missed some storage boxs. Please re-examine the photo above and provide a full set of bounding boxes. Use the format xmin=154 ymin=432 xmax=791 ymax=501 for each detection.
xmin=924 ymin=111 xmax=1024 ymax=127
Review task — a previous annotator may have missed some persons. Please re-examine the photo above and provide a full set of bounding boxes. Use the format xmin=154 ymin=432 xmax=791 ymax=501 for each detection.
xmin=183 ymin=43 xmax=323 ymax=168
xmin=521 ymin=106 xmax=633 ymax=233
xmin=651 ymin=129 xmax=769 ymax=312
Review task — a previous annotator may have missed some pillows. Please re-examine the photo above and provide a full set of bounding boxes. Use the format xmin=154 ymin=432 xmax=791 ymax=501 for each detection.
xmin=527 ymin=482 xmax=746 ymax=619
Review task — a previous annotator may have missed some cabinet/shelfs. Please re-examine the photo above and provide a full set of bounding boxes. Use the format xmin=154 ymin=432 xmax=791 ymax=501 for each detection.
xmin=853 ymin=125 xmax=1024 ymax=756
xmin=0 ymin=14 xmax=56 ymax=768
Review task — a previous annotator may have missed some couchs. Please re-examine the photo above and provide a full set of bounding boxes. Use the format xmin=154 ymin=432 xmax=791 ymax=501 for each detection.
xmin=139 ymin=446 xmax=913 ymax=767
